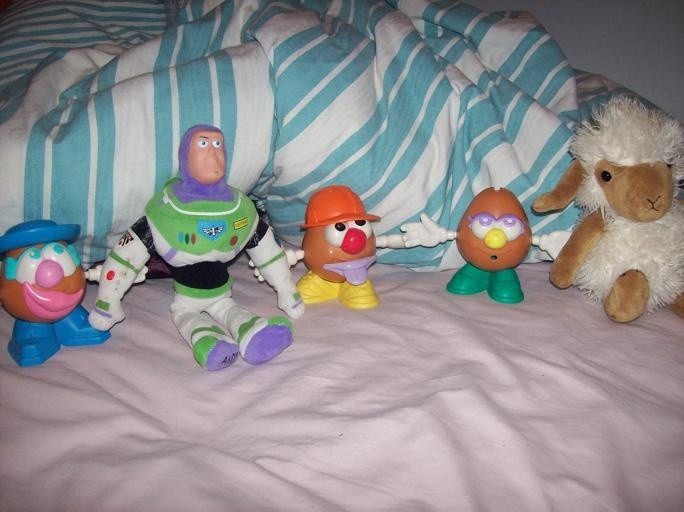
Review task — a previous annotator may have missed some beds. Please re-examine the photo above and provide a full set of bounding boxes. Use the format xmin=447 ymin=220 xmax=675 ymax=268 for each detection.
xmin=0 ymin=0 xmax=684 ymax=512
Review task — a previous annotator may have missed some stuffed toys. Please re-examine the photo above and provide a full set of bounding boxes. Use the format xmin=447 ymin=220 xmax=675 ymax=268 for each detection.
xmin=529 ymin=95 xmax=684 ymax=324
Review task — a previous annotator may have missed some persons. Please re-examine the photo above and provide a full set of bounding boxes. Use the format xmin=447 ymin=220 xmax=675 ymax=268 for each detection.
xmin=87 ymin=123 xmax=305 ymax=372
